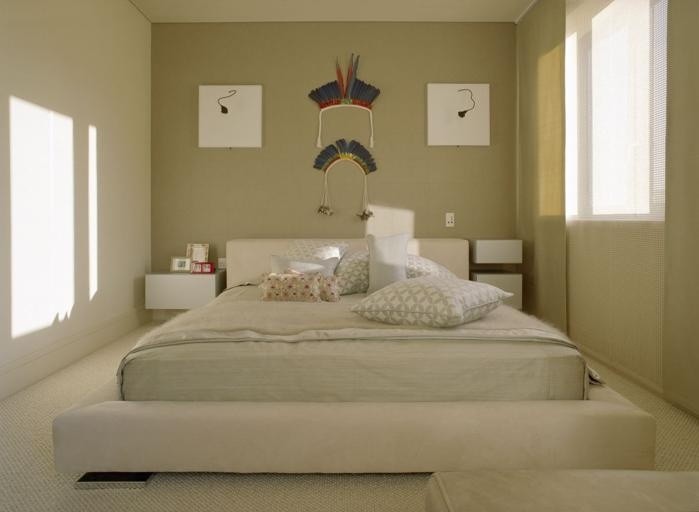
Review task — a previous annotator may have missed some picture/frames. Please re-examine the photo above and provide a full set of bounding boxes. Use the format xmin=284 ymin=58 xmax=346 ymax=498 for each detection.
xmin=170 ymin=242 xmax=209 ymax=273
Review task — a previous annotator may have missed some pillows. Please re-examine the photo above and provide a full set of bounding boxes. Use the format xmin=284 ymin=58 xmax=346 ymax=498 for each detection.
xmin=259 ymin=235 xmax=515 ymax=328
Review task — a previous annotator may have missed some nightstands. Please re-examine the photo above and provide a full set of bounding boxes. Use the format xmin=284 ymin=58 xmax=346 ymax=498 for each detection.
xmin=470 ymin=238 xmax=524 ymax=313
xmin=144 ymin=269 xmax=226 ymax=310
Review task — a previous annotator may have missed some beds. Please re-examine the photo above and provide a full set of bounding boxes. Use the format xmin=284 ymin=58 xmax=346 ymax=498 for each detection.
xmin=52 ymin=237 xmax=658 ymax=474
